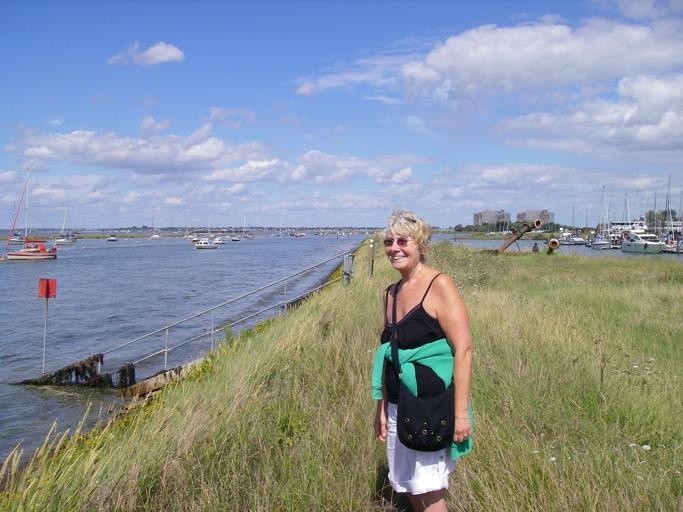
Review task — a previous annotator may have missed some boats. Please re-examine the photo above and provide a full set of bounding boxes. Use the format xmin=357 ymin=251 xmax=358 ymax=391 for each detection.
xmin=106 ymin=236 xmax=119 ymax=241
xmin=184 ymin=233 xmax=253 ymax=249
xmin=151 ymin=234 xmax=162 ymax=240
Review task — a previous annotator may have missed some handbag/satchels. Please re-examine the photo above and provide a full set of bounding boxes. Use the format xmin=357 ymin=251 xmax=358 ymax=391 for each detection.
xmin=395 ymin=380 xmax=456 ymax=452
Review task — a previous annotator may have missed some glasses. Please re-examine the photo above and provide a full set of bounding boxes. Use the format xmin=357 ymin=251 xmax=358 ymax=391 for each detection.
xmin=383 ymin=238 xmax=407 ymax=245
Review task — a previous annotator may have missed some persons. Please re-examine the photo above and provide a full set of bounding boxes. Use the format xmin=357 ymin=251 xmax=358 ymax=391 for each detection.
xmin=532 ymin=241 xmax=538 ymax=252
xmin=369 ymin=206 xmax=474 ymax=511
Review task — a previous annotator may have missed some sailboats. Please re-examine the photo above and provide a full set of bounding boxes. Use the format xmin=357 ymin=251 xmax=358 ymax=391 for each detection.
xmin=5 ymin=167 xmax=58 ymax=261
xmin=56 ymin=205 xmax=78 ymax=246
xmin=558 ymin=174 xmax=683 ymax=254
